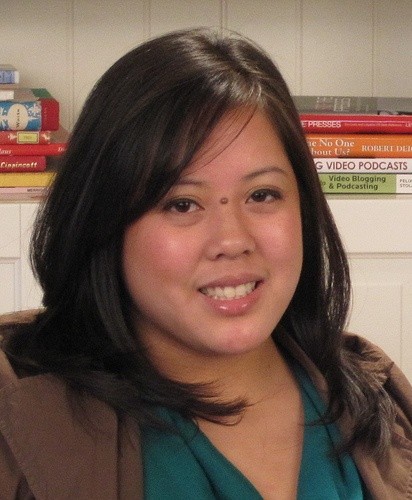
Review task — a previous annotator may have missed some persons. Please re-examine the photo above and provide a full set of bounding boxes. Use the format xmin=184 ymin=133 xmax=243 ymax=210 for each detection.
xmin=0 ymin=26 xmax=412 ymax=500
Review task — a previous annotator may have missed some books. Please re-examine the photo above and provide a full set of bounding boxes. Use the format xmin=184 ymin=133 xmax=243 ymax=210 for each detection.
xmin=0 ymin=63 xmax=71 ymax=194
xmin=290 ymin=95 xmax=412 ymax=194
